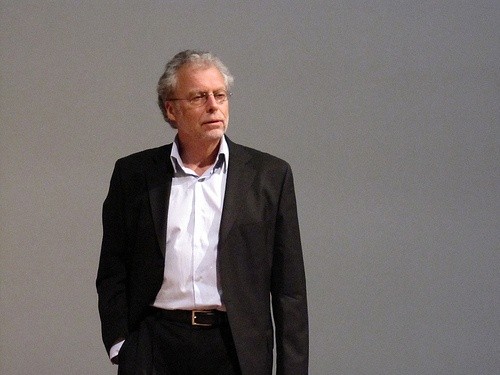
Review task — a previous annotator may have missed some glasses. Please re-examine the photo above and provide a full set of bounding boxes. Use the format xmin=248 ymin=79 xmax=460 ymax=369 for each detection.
xmin=166 ymin=89 xmax=233 ymax=105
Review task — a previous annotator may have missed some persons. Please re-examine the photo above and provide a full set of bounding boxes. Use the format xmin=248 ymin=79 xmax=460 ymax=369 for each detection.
xmin=95 ymin=48 xmax=310 ymax=375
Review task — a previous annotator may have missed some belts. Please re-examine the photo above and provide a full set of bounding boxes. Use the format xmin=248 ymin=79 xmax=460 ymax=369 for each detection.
xmin=152 ymin=306 xmax=228 ymax=329
